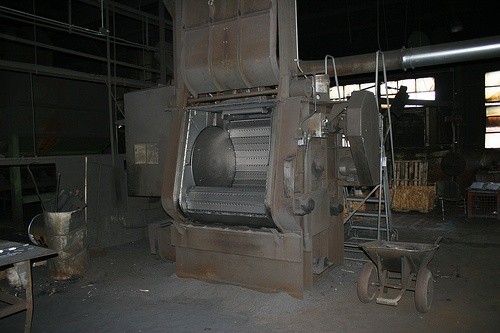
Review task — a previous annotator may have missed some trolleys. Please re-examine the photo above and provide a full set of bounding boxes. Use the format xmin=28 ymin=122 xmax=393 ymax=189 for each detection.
xmin=355 ymin=239 xmax=443 ymax=314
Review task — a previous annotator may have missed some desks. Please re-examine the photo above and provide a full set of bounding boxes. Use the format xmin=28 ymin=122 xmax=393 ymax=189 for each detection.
xmin=0 ymin=241 xmax=59 ymax=333
